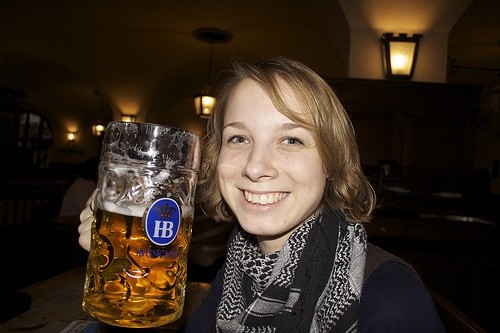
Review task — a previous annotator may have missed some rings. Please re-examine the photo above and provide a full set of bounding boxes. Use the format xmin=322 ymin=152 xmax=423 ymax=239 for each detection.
xmin=89 ymin=202 xmax=94 ymax=211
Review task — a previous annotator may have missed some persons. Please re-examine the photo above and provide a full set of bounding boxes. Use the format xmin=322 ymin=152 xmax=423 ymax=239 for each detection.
xmin=55 ymin=156 xmax=101 ymax=265
xmin=452 ymin=159 xmax=500 ymax=223
xmin=77 ymin=56 xmax=444 ymax=333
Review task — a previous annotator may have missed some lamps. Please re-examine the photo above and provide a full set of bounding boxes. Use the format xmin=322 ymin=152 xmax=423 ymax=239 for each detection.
xmin=379 ymin=31 xmax=424 ymax=79
xmin=89 ymin=90 xmax=107 ymax=140
xmin=191 ymin=26 xmax=233 ymax=119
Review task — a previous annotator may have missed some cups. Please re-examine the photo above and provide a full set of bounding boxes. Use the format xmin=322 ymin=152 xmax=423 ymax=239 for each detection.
xmin=82 ymin=121 xmax=201 ymax=328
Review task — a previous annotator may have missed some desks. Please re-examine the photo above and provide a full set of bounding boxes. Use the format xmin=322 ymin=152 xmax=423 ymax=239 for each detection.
xmin=0 ymin=264 xmax=212 ymax=333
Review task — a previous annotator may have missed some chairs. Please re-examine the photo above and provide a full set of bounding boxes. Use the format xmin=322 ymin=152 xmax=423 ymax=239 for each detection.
xmin=46 ymin=215 xmax=90 ymax=277
xmin=360 ymin=161 xmax=499 ymax=332
